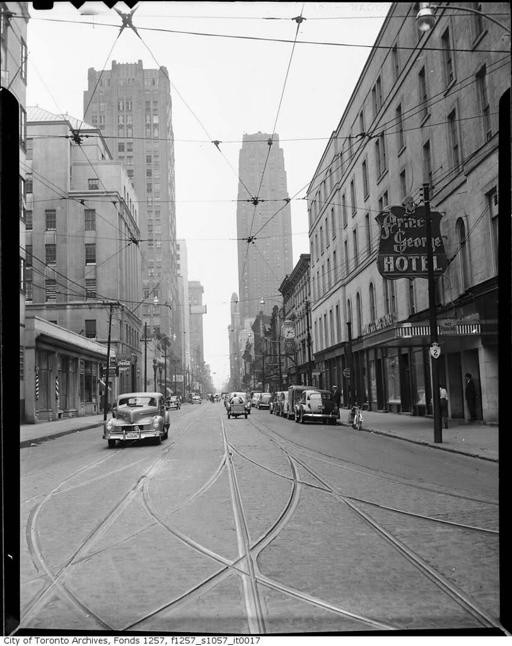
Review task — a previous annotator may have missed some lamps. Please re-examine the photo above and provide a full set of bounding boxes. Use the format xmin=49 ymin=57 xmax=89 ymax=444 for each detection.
xmin=414 ymin=0 xmax=512 ymax=34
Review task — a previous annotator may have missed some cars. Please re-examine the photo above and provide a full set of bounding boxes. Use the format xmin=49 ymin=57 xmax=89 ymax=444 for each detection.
xmin=104 ymin=392 xmax=171 ymax=445
xmin=249 ymin=384 xmax=341 ymax=424
xmin=224 ymin=391 xmax=250 ymax=415
xmin=164 ymin=392 xmax=202 ymax=412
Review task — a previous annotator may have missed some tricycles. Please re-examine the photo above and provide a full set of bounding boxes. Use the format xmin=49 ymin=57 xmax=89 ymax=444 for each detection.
xmin=226 ymin=396 xmax=250 ymax=419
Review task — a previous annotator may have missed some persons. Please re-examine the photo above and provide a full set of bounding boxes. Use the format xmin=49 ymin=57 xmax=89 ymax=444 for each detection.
xmin=229 ymin=393 xmax=243 ymax=405
xmin=332 ymin=384 xmax=341 ymax=420
xmin=464 ymin=372 xmax=478 ymax=422
xmin=439 ymin=385 xmax=450 ymax=430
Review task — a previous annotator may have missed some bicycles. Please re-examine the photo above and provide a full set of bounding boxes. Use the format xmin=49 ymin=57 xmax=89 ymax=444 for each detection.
xmin=347 ymin=401 xmax=364 ymax=431
